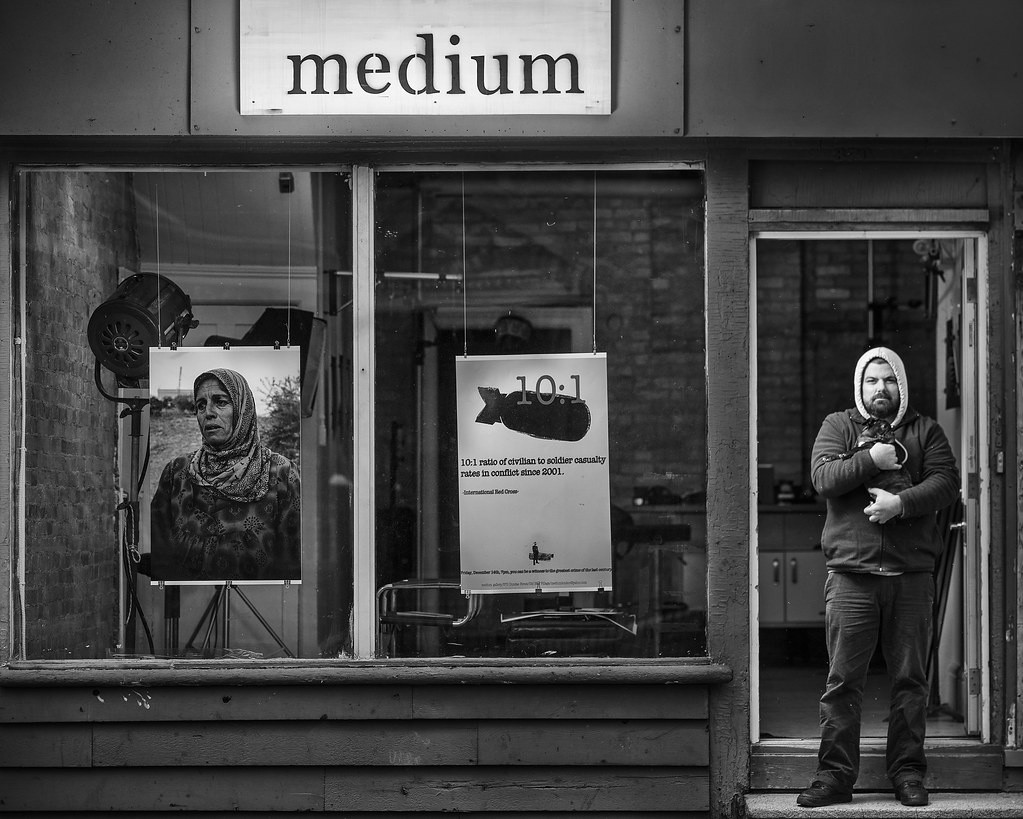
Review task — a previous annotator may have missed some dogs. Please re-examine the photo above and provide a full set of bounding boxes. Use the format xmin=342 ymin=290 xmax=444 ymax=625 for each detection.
xmin=820 ymin=416 xmax=917 ymax=523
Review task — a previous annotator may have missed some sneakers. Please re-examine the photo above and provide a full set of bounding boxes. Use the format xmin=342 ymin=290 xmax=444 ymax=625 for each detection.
xmin=796 ymin=780 xmax=852 ymax=807
xmin=897 ymin=779 xmax=928 ymax=806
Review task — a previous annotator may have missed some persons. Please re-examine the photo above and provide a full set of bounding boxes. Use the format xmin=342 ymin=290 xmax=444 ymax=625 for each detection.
xmin=796 ymin=346 xmax=961 ymax=807
xmin=151 ymin=369 xmax=302 ymax=580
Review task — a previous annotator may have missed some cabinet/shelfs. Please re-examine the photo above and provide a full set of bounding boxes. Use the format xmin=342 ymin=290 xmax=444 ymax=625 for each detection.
xmin=759 ymin=552 xmax=824 ymax=621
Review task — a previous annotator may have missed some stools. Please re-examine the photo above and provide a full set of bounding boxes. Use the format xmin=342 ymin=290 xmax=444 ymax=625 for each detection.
xmin=620 ymin=523 xmax=695 ymax=658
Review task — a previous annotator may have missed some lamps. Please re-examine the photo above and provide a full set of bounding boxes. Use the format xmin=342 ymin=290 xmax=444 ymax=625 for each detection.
xmin=87 ymin=273 xmax=200 ymax=661
